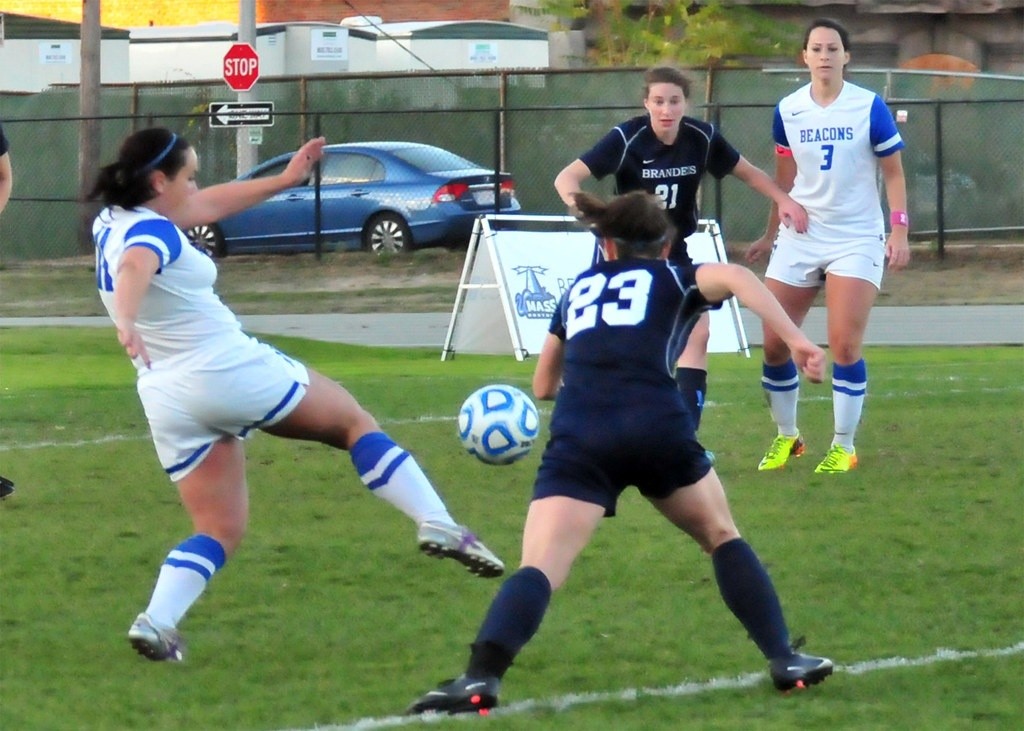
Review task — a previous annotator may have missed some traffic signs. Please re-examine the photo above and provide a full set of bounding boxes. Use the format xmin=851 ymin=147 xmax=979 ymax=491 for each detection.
xmin=209 ymin=101 xmax=274 ymax=127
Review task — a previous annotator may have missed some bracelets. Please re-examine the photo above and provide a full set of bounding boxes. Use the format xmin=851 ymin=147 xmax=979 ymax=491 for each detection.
xmin=890 ymin=210 xmax=909 ymax=226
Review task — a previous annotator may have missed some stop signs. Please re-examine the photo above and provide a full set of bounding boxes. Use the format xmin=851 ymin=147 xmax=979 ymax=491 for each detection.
xmin=222 ymin=43 xmax=259 ymax=92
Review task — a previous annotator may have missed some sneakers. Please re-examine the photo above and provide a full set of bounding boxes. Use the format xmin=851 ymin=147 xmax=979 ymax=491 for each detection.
xmin=814 ymin=443 xmax=858 ymax=474
xmin=770 ymin=636 xmax=832 ymax=694
xmin=405 ymin=665 xmax=499 ymax=715
xmin=757 ymin=427 xmax=803 ymax=470
xmin=128 ymin=611 xmax=186 ymax=663
xmin=417 ymin=520 xmax=504 ymax=578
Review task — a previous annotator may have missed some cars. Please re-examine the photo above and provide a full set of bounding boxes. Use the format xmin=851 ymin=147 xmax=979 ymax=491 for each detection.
xmin=184 ymin=142 xmax=520 ymax=258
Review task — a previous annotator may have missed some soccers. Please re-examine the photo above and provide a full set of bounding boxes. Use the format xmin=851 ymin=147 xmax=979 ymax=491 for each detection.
xmin=456 ymin=383 xmax=541 ymax=468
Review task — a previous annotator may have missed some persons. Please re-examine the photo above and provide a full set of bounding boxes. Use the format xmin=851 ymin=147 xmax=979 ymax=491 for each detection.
xmin=0 ymin=123 xmax=16 ymax=499
xmin=87 ymin=126 xmax=505 ymax=664
xmin=746 ymin=16 xmax=912 ymax=474
xmin=405 ymin=190 xmax=833 ymax=717
xmin=555 ymin=67 xmax=810 ymax=467
